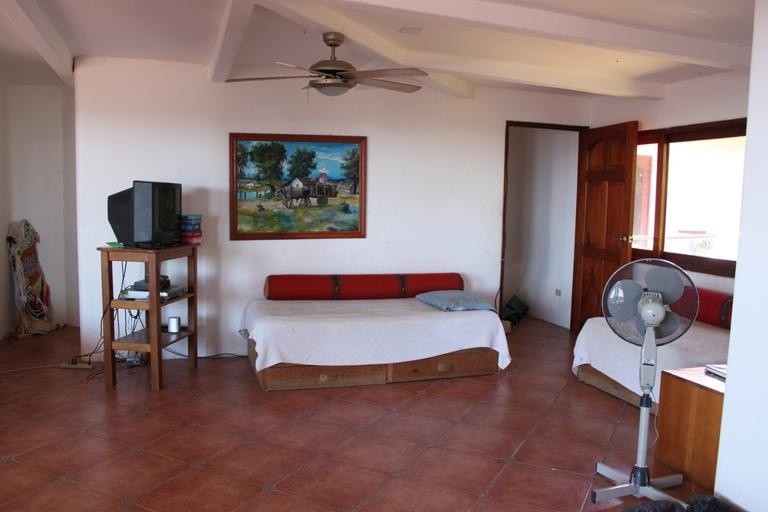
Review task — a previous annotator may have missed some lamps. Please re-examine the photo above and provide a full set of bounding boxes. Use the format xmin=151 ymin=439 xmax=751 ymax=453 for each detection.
xmin=309 ymin=79 xmax=356 ymax=96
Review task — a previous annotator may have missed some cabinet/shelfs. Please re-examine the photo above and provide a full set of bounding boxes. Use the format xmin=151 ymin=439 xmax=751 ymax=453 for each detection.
xmin=97 ymin=243 xmax=201 ymax=391
xmin=655 ymin=366 xmax=724 ymax=493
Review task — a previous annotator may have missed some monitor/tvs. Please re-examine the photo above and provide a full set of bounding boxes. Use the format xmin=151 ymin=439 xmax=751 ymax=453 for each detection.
xmin=107 ymin=180 xmax=183 ymax=249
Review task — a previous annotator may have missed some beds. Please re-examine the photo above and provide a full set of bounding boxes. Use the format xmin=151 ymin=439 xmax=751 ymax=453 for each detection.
xmin=572 ymin=313 xmax=730 ymax=417
xmin=238 ymin=297 xmax=513 ymax=392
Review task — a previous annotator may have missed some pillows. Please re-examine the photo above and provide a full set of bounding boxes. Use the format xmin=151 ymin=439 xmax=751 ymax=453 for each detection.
xmin=264 ymin=273 xmax=464 ymax=300
xmin=415 ymin=289 xmax=498 ymax=313
xmin=669 ymin=285 xmax=733 ymax=329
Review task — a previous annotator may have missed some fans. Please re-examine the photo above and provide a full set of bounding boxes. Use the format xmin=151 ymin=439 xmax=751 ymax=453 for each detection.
xmin=225 ymin=32 xmax=428 ymax=94
xmin=590 ymin=257 xmax=700 ymax=511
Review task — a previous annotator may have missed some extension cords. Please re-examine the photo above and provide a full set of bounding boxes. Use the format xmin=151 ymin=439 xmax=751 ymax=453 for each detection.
xmin=59 ymin=363 xmax=93 ymax=369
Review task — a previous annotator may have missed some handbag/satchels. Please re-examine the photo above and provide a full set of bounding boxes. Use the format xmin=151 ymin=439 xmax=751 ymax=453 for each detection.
xmin=501 ymin=294 xmax=529 ymax=327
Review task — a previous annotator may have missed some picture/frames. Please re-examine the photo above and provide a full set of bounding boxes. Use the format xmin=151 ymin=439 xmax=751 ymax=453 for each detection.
xmin=229 ymin=133 xmax=367 ymax=240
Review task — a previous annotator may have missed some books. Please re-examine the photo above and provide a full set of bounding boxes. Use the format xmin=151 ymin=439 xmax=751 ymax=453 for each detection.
xmin=704 ymin=363 xmax=728 ymax=379
xmin=181 ymin=213 xmax=204 ymax=246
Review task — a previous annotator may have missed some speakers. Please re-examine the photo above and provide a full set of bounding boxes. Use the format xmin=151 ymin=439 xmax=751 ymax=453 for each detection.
xmin=167 ymin=316 xmax=181 ymax=334
